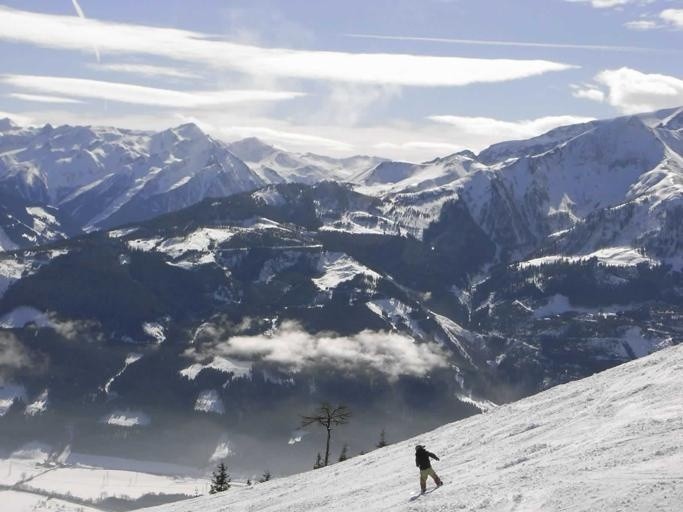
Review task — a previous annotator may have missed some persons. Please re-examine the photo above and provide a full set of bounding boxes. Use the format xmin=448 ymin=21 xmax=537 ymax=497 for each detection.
xmin=413 ymin=443 xmax=443 ymax=492
